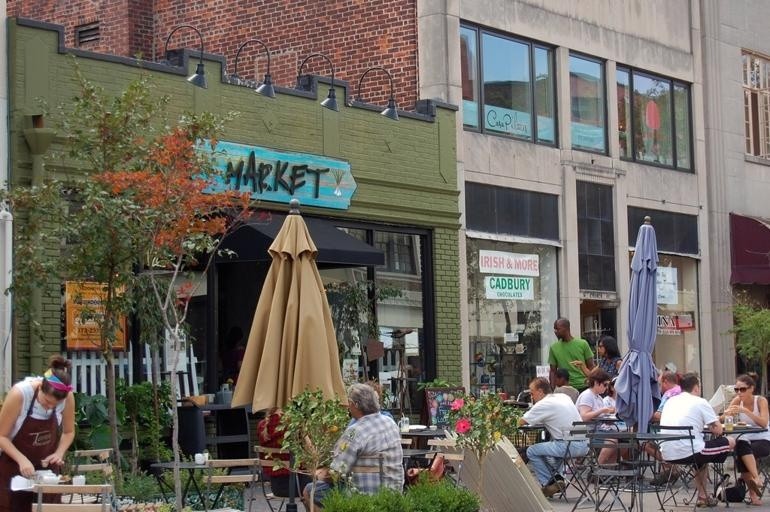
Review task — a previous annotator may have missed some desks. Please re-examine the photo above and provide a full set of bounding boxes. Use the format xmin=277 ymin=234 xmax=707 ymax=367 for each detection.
xmin=400 ymin=427 xmax=449 ymax=437
xmin=176 ymin=406 xmax=251 ymax=470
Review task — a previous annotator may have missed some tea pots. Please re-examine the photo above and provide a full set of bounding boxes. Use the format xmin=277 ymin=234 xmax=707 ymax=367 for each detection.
xmin=37 ymin=469 xmax=62 ymax=485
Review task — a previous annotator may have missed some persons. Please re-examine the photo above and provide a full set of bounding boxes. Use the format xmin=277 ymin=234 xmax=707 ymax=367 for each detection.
xmin=548 ymin=319 xmax=593 ymax=393
xmin=257 ymin=408 xmax=312 ymax=498
xmin=0 ymin=354 xmax=75 ymax=512
xmin=655 ymin=373 xmax=735 ymax=507
xmin=723 ymin=373 xmax=770 ymax=507
xmin=346 ymin=381 xmax=393 ymax=427
xmin=303 ymin=383 xmax=406 ymax=512
xmin=220 ymin=324 xmax=246 ymax=458
xmin=553 ymin=336 xmax=623 ymax=484
xmin=521 ymin=377 xmax=589 ymax=498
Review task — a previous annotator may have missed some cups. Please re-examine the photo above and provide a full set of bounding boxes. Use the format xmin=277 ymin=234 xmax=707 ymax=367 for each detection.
xmin=430 ymin=425 xmax=437 ymax=431
xmin=725 ymin=416 xmax=734 ymax=432
xmin=398 ymin=417 xmax=409 ymax=433
xmin=41 ymin=460 xmax=50 ymax=467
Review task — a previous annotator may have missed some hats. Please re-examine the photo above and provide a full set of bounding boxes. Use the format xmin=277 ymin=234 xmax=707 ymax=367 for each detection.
xmin=552 ymin=368 xmax=569 ymax=379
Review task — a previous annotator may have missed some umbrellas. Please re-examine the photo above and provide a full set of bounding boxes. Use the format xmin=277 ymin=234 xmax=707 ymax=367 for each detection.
xmin=230 ymin=198 xmax=350 ymax=512
xmin=613 ymin=215 xmax=661 ymax=455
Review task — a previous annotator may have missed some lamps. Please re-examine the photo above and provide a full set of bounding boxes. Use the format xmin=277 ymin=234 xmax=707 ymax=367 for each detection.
xmin=164 ymin=25 xmax=208 ymax=89
xmin=235 ymin=39 xmax=276 ymax=98
xmin=358 ymin=68 xmax=399 ymax=120
xmin=299 ymin=54 xmax=339 ymax=112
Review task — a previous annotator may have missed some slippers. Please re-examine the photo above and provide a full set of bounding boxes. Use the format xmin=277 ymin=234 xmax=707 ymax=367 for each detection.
xmin=750 ymin=479 xmax=762 ymax=497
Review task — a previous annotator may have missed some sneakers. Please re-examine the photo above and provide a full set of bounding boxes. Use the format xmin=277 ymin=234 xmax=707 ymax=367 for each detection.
xmin=539 ymin=473 xmax=565 ymax=497
xmin=697 ymin=496 xmax=718 ymax=507
xmin=650 ymin=471 xmax=676 ymax=486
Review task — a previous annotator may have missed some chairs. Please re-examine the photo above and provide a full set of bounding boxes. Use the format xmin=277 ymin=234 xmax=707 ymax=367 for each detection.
xmin=32 ymin=438 xmax=465 ymax=512
xmin=541 ymin=411 xmax=770 ymax=512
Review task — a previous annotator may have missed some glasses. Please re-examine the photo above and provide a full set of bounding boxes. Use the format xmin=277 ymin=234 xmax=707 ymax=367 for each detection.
xmin=604 ymin=384 xmax=607 ymax=387
xmin=734 ymin=388 xmax=746 ymax=392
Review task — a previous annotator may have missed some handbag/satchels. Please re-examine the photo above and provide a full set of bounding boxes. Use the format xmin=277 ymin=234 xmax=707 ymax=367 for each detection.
xmin=407 ymin=452 xmax=445 ymax=484
xmin=713 ymin=474 xmax=746 ymax=503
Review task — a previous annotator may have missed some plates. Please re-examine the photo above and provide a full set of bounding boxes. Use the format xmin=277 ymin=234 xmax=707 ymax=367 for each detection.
xmin=408 ymin=425 xmax=427 ymax=432
